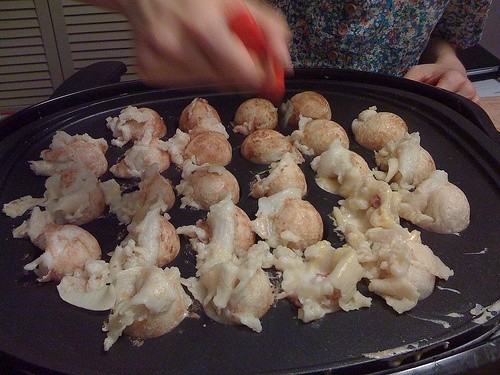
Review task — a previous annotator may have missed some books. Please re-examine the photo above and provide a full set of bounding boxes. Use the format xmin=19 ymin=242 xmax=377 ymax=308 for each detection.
xmin=460 ymin=42 xmax=500 ymax=76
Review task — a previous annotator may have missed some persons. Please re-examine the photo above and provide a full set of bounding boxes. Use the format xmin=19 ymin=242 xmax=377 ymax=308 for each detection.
xmin=70 ymin=0 xmax=492 ymax=105
